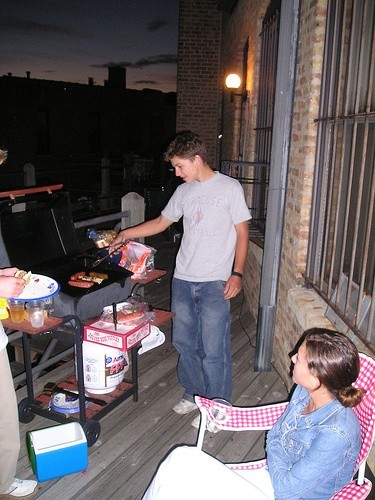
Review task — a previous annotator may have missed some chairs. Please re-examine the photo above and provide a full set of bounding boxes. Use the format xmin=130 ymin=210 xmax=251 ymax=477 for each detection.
xmin=194 ymin=352 xmax=375 ymax=500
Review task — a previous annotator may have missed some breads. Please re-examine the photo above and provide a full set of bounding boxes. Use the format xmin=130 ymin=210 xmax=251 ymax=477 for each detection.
xmin=96 ymin=231 xmax=117 ymax=248
xmin=14 ymin=270 xmax=32 ymax=284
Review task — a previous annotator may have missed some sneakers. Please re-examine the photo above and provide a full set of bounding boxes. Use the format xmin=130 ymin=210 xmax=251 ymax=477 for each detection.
xmin=191 ymin=413 xmax=207 ymax=431
xmin=172 ymin=399 xmax=198 ymax=414
xmin=1 ymin=478 xmax=38 ymax=497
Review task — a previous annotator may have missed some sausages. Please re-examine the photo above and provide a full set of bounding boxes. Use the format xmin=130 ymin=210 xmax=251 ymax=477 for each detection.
xmin=68 ymin=280 xmax=91 ymax=288
xmin=70 ymin=272 xmax=85 ymax=280
xmin=105 ymin=308 xmax=144 ymax=323
xmin=89 ymin=272 xmax=108 ymax=279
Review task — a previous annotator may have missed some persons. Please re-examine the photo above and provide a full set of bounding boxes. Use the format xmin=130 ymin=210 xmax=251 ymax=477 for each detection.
xmin=109 ymin=130 xmax=253 ymax=430
xmin=0 ymin=268 xmax=39 ymax=497
xmin=0 ymin=150 xmax=11 ymax=268
xmin=141 ymin=327 xmax=367 ymax=500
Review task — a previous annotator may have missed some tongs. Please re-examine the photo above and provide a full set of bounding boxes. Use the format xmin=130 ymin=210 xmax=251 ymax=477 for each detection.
xmin=88 ymin=241 xmax=129 ymax=270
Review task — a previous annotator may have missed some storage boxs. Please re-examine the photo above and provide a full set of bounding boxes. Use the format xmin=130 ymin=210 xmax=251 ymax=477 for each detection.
xmin=26 ymin=421 xmax=88 ymax=483
xmin=84 ymin=319 xmax=153 ymax=352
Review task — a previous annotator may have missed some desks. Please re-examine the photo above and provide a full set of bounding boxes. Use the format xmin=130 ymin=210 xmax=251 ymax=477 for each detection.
xmin=70 ymin=193 xmax=123 ymax=222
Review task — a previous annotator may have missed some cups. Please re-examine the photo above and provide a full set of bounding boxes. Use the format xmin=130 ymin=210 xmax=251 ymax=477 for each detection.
xmin=207 ymin=398 xmax=233 ymax=433
xmin=25 ymin=300 xmax=45 ymax=327
xmin=7 ymin=299 xmax=25 ymax=323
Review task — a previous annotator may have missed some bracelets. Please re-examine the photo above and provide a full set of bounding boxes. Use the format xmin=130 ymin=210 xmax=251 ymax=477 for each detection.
xmin=231 ymin=271 xmax=243 ymax=279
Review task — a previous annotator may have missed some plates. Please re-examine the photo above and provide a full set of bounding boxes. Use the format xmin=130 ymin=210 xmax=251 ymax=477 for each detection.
xmin=9 ymin=274 xmax=60 ymax=301
xmin=52 ymin=391 xmax=90 ymax=414
